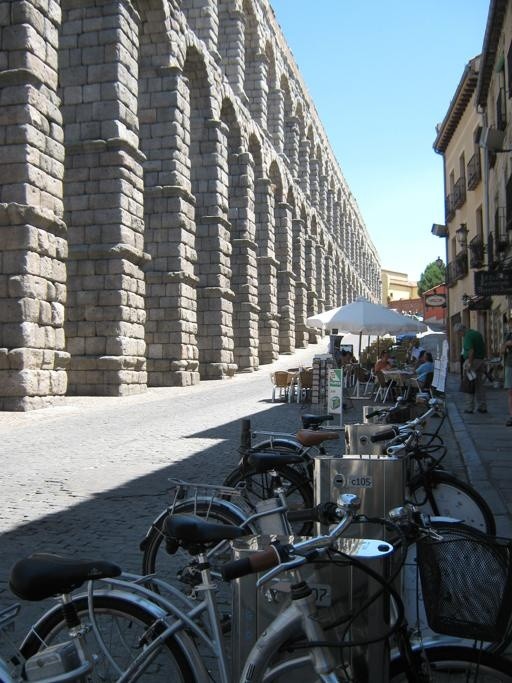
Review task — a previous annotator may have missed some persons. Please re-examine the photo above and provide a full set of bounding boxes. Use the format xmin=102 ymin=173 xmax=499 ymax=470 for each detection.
xmin=336 ymin=349 xmax=358 ymax=375
xmin=452 ymin=322 xmax=489 ymax=413
xmin=499 ymin=316 xmax=511 ymax=426
xmin=414 ymin=349 xmax=429 ymax=370
xmin=373 ymin=350 xmax=399 ymax=387
xmin=405 ymin=350 xmax=434 ymax=394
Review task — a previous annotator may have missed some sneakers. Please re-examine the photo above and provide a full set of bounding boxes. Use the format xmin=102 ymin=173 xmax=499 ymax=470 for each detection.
xmin=464 ymin=409 xmax=487 ymax=413
xmin=506 ymin=417 xmax=512 ymax=426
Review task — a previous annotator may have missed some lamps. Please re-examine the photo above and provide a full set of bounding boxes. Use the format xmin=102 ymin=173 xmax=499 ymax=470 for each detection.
xmin=461 ymin=293 xmax=484 ymax=306
xmin=455 ymin=222 xmax=484 ymax=250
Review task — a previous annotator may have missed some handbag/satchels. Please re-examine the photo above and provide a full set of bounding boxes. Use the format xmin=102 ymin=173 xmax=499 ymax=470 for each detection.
xmin=460 ymin=377 xmax=475 ymax=393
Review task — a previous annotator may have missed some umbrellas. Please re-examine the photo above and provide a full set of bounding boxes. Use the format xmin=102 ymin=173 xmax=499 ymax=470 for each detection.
xmin=304 ymin=295 xmax=428 ymax=359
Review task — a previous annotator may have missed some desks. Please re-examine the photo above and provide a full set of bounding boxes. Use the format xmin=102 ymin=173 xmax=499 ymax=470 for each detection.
xmin=482 ymin=356 xmax=502 ymax=383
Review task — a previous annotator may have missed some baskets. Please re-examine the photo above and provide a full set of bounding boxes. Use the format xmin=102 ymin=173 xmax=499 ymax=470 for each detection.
xmin=415 ymin=529 xmax=512 ymax=643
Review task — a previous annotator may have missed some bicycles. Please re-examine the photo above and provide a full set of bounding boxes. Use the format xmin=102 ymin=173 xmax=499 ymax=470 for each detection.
xmin=0 ymin=396 xmax=512 ymax=683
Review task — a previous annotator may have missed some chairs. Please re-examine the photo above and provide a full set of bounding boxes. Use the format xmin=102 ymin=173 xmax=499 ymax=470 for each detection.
xmin=270 ymin=335 xmax=438 ymax=403
